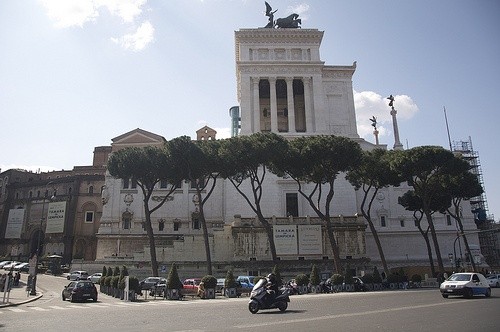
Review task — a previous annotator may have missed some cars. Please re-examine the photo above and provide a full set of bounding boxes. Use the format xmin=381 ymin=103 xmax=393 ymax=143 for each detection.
xmin=86 ymin=272 xmax=102 ymax=285
xmin=66 ymin=270 xmax=89 ymax=281
xmin=0 ymin=260 xmax=44 ymax=273
xmin=62 ymin=280 xmax=98 ymax=303
xmin=138 ymin=276 xmax=259 ymax=296
xmin=482 ymin=272 xmax=500 ymax=288
xmin=439 ymin=272 xmax=491 ymax=299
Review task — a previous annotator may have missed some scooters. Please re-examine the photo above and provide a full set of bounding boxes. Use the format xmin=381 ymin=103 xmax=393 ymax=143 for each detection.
xmin=248 ymin=277 xmax=293 ymax=314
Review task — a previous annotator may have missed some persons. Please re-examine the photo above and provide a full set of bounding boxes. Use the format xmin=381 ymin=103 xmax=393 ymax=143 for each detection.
xmin=265 ymin=273 xmax=278 ymax=306
xmin=269 ymin=9 xmax=278 ymax=25
xmin=370 ymin=116 xmax=377 ymax=130
xmin=387 ymin=95 xmax=395 ymax=108
xmin=13 ymin=272 xmax=20 ymax=286
